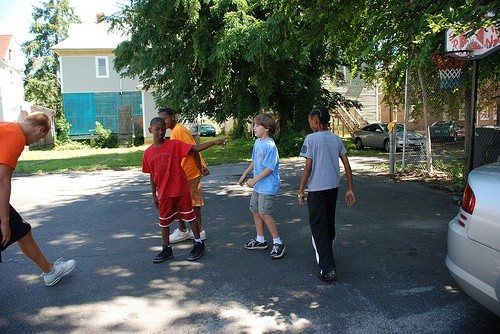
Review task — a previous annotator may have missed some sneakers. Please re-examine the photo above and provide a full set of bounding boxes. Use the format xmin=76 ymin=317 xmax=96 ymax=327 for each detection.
xmin=42 ymin=259 xmax=76 ymax=286
xmin=188 ymin=240 xmax=204 ymax=259
xmin=168 ymin=227 xmax=190 ymax=243
xmin=270 ymin=243 xmax=285 ymax=258
xmin=243 ymin=239 xmax=268 ymax=249
xmin=189 ymin=229 xmax=206 ymax=240
xmin=152 ymin=245 xmax=173 ymax=261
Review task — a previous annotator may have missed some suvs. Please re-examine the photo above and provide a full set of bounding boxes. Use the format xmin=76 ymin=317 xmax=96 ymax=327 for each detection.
xmin=430 ymin=120 xmax=465 ymax=142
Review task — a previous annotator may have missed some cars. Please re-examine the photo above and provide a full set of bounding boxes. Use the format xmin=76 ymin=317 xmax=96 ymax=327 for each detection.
xmin=197 ymin=124 xmax=216 ymax=137
xmin=351 ymin=122 xmax=425 ymax=153
xmin=445 ymin=160 xmax=500 ymax=318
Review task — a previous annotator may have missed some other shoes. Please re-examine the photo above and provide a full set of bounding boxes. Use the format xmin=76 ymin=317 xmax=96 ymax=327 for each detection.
xmin=319 ymin=269 xmax=336 ymax=280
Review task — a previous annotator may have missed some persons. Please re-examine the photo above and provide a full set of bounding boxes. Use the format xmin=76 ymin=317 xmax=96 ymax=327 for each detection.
xmin=142 ymin=117 xmax=230 ymax=262
xmin=157 ymin=107 xmax=210 ymax=243
xmin=0 ymin=112 xmax=76 ymax=287
xmin=297 ymin=106 xmax=356 ymax=281
xmin=239 ymin=113 xmax=287 ymax=258
